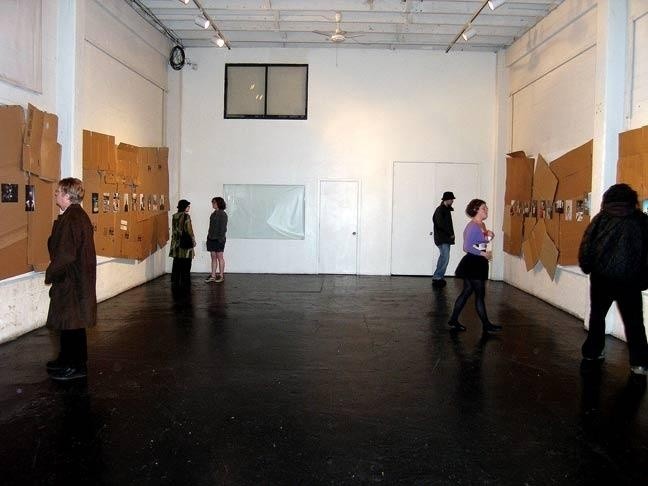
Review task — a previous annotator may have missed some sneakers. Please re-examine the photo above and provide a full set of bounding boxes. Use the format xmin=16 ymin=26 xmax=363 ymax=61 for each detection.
xmin=47 ymin=357 xmax=63 ymax=371
xmin=433 ymin=279 xmax=446 ymax=285
xmin=50 ymin=367 xmax=86 ymax=379
xmin=583 ymin=352 xmax=607 ymax=361
xmin=448 ymin=320 xmax=465 ymax=331
xmin=483 ymin=325 xmax=502 ymax=333
xmin=215 ymin=277 xmax=224 ymax=283
xmin=630 ymin=365 xmax=644 ymax=374
xmin=205 ymin=274 xmax=214 ymax=282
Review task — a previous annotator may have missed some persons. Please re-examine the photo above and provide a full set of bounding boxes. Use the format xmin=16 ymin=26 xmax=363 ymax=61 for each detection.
xmin=169 ymin=199 xmax=197 ymax=295
xmin=205 ymin=196 xmax=228 ymax=283
xmin=3 ymin=184 xmax=16 ymax=200
xmin=448 ymin=198 xmax=504 ymax=333
xmin=43 ymin=177 xmax=99 ymax=382
xmin=578 ymin=183 xmax=647 ymax=376
xmin=25 ymin=188 xmax=34 ymax=211
xmin=510 ymin=193 xmax=590 ymax=221
xmin=431 ymin=191 xmax=457 ymax=282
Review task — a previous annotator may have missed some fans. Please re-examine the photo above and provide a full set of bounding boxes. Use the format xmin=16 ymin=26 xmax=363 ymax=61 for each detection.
xmin=312 ymin=12 xmax=368 ymax=46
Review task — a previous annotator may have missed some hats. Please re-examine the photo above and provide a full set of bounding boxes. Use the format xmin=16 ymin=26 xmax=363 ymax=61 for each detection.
xmin=441 ymin=192 xmax=455 ymax=200
xmin=177 ymin=199 xmax=190 ymax=209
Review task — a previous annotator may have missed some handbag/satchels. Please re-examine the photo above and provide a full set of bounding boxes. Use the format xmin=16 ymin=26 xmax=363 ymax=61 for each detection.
xmin=180 ymin=230 xmax=193 ymax=249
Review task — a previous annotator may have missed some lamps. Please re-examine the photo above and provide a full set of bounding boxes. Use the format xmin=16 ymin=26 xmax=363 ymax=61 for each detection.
xmin=461 ymin=0 xmax=508 ymax=41
xmin=177 ymin=0 xmax=232 ymax=49
